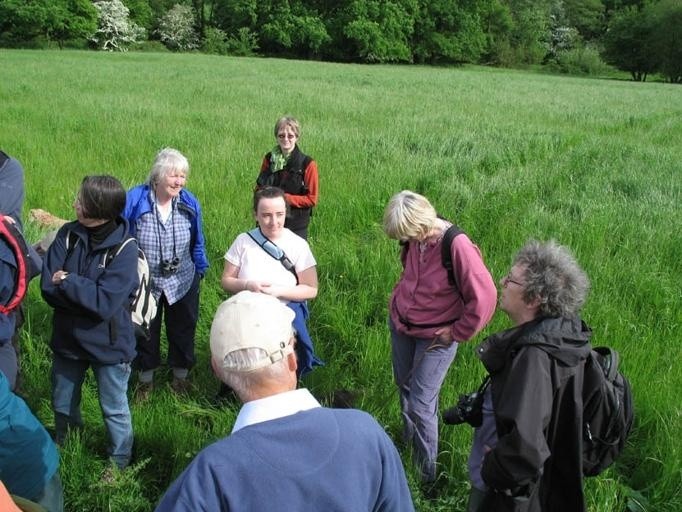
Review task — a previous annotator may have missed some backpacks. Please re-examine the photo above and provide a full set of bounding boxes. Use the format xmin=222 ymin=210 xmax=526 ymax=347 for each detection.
xmin=107 ymin=233 xmax=159 ymax=345
xmin=583 ymin=345 xmax=634 ymax=479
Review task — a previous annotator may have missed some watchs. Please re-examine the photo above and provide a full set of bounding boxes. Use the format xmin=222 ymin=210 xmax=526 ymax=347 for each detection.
xmin=58 ymin=272 xmax=72 ymax=284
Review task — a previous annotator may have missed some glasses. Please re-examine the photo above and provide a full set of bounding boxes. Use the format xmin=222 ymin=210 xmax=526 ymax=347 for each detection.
xmin=277 ymin=134 xmax=295 ymax=139
xmin=503 ymin=275 xmax=525 ymax=287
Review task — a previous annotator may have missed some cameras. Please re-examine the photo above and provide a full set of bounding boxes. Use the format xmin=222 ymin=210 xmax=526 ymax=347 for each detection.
xmin=440 ymin=392 xmax=483 ymax=427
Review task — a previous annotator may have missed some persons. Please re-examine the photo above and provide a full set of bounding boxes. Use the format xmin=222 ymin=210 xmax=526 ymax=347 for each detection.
xmin=124 ymin=146 xmax=212 ymax=407
xmin=152 ymin=288 xmax=418 ymax=511
xmin=442 ymin=235 xmax=636 ymax=512
xmin=216 ymin=185 xmax=321 ymax=398
xmin=379 ymin=186 xmax=499 ymax=505
xmin=252 ymin=115 xmax=320 ymax=244
xmin=1 ymin=369 xmax=64 ymax=512
xmin=0 ymin=144 xmax=27 ymax=243
xmin=37 ymin=173 xmax=142 ymax=486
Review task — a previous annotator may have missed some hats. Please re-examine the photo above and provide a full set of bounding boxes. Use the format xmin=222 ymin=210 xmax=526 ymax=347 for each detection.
xmin=209 ymin=289 xmax=297 ymax=372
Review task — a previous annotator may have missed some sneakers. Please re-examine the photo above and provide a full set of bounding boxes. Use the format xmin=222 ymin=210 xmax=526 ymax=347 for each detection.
xmin=100 ymin=465 xmax=122 ymax=484
xmin=135 ymin=382 xmax=153 ymax=405
xmin=173 ymin=377 xmax=191 ymax=396
